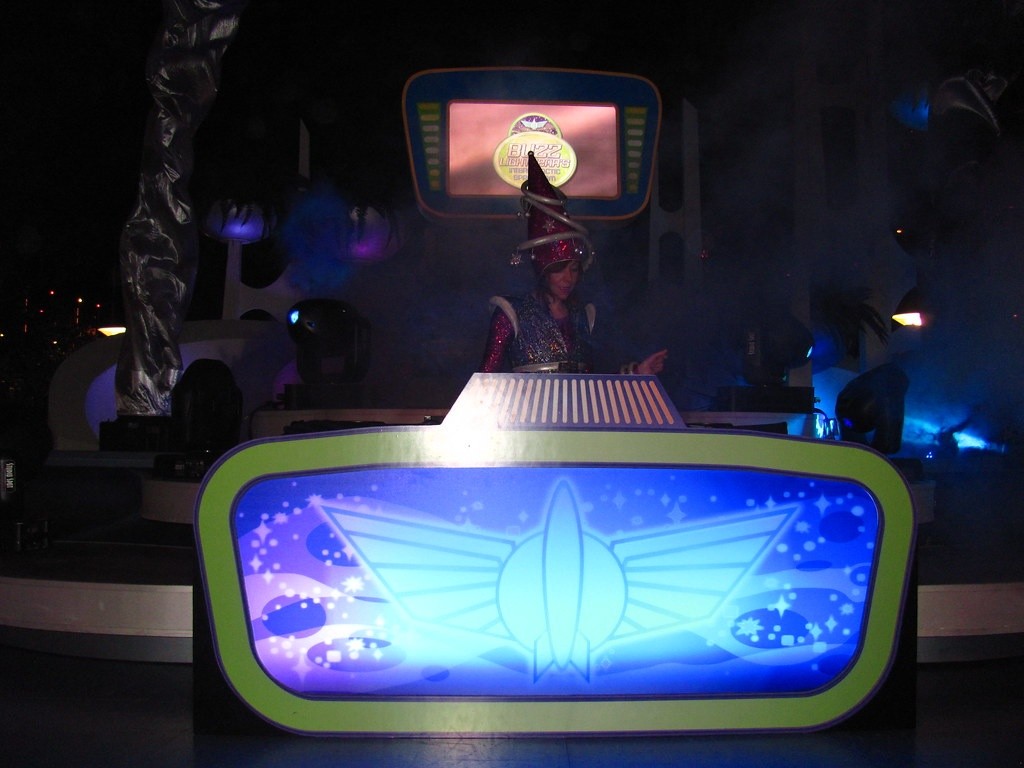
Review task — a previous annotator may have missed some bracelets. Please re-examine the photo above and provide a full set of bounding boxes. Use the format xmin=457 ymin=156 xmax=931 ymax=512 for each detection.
xmin=620 ymin=365 xmax=629 ymax=375
xmin=628 ymin=362 xmax=639 ymax=374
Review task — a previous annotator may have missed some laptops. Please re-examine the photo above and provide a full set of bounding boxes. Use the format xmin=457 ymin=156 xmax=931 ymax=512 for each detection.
xmin=718 ymin=385 xmax=814 ymax=414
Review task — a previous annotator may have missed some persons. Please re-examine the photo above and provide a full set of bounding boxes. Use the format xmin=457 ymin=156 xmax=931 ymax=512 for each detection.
xmin=478 ymin=150 xmax=667 ymax=376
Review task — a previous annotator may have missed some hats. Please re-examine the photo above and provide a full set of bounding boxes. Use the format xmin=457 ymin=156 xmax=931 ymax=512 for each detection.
xmin=509 ymin=151 xmax=595 ymax=273
